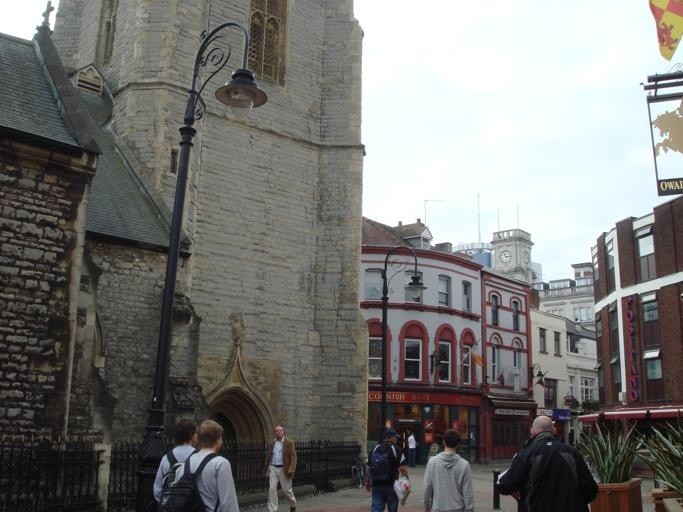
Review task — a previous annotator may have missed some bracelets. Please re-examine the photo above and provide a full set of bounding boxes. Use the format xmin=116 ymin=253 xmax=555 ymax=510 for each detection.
xmin=364 ymin=479 xmax=370 ymax=483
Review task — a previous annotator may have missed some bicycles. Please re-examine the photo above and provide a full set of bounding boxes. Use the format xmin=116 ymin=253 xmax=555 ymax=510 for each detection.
xmin=351 ymin=457 xmax=370 ymax=489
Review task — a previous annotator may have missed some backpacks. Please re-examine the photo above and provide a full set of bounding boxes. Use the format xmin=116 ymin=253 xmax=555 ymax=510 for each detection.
xmin=369 ymin=444 xmax=402 ymax=483
xmin=161 ymin=450 xmax=197 ymax=505
xmin=167 ymin=452 xmax=219 ymax=512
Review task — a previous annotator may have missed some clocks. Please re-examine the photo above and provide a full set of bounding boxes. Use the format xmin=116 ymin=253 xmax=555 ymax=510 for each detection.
xmin=521 ymin=250 xmax=529 ymax=265
xmin=499 ymin=249 xmax=512 ymax=265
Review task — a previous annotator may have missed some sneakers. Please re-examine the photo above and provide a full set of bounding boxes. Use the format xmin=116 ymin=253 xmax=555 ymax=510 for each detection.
xmin=290 ymin=507 xmax=296 ymax=512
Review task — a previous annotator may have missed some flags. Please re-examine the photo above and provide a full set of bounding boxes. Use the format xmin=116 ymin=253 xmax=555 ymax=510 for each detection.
xmin=648 ymin=0 xmax=682 ymax=63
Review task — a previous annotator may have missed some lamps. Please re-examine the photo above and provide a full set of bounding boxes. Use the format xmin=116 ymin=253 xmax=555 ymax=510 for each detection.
xmin=428 ymin=350 xmax=441 ymax=376
xmin=528 ymin=362 xmax=545 ymax=385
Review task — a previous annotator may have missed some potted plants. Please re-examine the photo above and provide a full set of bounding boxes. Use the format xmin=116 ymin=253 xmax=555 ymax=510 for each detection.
xmin=569 ymin=408 xmax=648 ymax=512
xmin=623 ymin=405 xmax=682 ymax=511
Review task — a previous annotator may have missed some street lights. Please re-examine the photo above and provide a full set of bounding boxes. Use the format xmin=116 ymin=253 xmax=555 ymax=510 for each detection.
xmin=377 ymin=245 xmax=427 ymax=445
xmin=128 ymin=23 xmax=268 ymax=512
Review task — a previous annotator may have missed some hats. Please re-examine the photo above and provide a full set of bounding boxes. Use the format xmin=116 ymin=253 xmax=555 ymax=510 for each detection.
xmin=384 ymin=428 xmax=400 ymax=439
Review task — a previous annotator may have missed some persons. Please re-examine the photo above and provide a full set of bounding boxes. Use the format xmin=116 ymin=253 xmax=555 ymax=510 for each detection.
xmin=152 ymin=419 xmax=199 ymax=507
xmin=364 ymin=428 xmax=410 ymax=511
xmin=494 ymin=415 xmax=598 ymax=511
xmin=262 ymin=424 xmax=298 ymax=511
xmin=421 ymin=428 xmax=474 ymax=512
xmin=406 ymin=431 xmax=418 ymax=468
xmin=174 ymin=418 xmax=240 ymax=511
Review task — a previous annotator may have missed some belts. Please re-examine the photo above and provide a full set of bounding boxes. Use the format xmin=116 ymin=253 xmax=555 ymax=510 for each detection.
xmin=273 ymin=465 xmax=284 ymax=467
